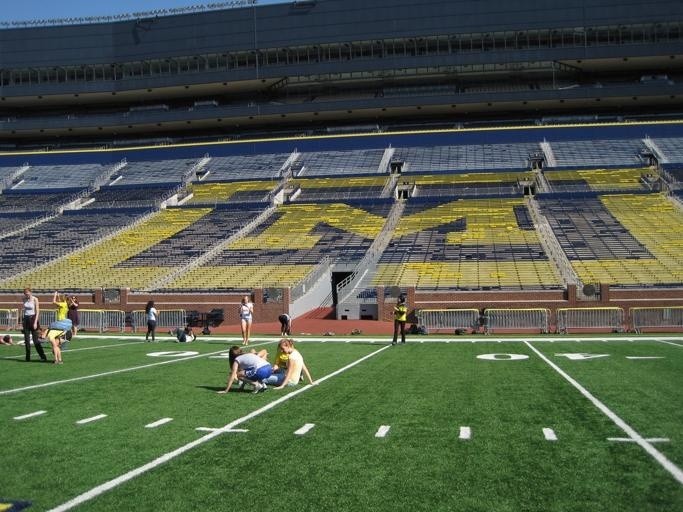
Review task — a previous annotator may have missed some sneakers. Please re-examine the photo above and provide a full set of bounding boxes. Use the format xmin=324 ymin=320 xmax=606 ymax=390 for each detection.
xmin=281 ymin=332 xmax=288 ymax=336
xmin=242 ymin=340 xmax=249 ymax=346
xmin=39 ymin=354 xmax=64 ymax=365
xmin=392 ymin=341 xmax=405 ymax=345
xmin=145 ymin=340 xmax=156 ymax=343
xmin=251 ymin=382 xmax=268 ymax=393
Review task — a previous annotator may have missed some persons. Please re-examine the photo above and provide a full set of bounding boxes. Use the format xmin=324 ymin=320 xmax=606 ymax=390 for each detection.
xmin=176 ymin=331 xmax=196 ymax=343
xmin=238 ymin=296 xmax=253 ymax=346
xmin=0 ymin=335 xmax=13 ymax=345
xmin=145 ymin=301 xmax=159 ymax=342
xmin=250 ymin=349 xmax=267 ymax=360
xmin=272 ymin=339 xmax=319 ymax=390
xmin=39 ymin=326 xmax=72 ymax=365
xmin=67 ymin=296 xmax=79 ymax=335
xmin=216 ymin=346 xmax=272 ymax=394
xmin=21 ymin=288 xmax=46 ymax=362
xmin=392 ymin=295 xmax=407 ymax=345
xmin=279 ymin=314 xmax=291 ymax=336
xmin=52 ymin=291 xmax=69 ymax=321
